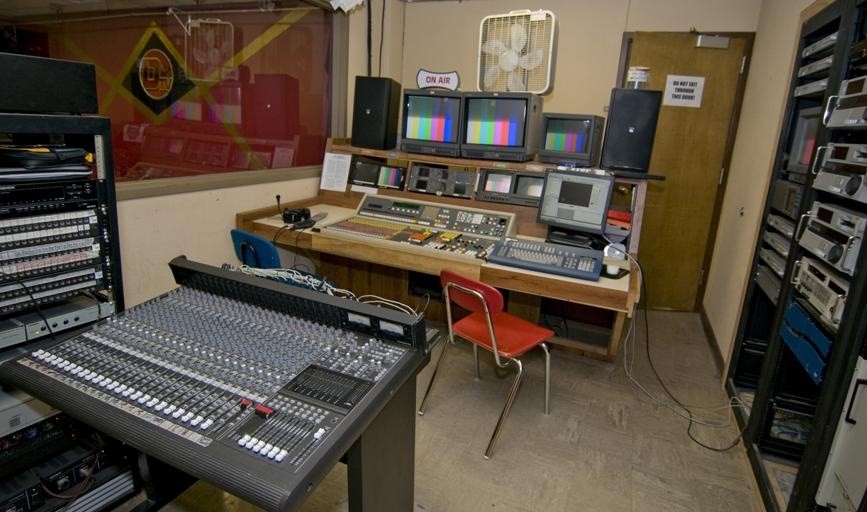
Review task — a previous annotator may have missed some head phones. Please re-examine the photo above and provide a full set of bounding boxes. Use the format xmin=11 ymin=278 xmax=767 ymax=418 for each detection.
xmin=283 ymin=207 xmax=311 ymax=223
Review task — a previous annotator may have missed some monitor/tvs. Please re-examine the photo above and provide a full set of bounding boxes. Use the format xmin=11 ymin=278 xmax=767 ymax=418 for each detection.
xmin=167 ymin=79 xmax=254 ymax=137
xmin=538 ymin=113 xmax=605 ymax=166
xmin=461 ymin=92 xmax=543 ymax=163
xmin=400 ymin=88 xmax=464 ymax=158
xmin=348 ymin=155 xmax=386 ymax=188
xmin=536 ymin=168 xmax=616 ymax=248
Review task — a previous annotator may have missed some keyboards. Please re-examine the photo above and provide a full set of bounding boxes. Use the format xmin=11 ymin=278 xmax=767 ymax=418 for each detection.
xmin=488 ymin=236 xmax=604 ymax=281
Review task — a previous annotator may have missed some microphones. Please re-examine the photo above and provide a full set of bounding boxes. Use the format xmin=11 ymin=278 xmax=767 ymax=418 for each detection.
xmin=276 ymin=195 xmax=284 ymax=217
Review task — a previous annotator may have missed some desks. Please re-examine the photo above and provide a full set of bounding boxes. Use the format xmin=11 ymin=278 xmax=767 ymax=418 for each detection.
xmin=236 ymin=197 xmax=642 ymax=364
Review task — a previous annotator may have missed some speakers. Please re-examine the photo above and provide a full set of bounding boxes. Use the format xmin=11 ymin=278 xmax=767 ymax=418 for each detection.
xmin=351 ymin=76 xmax=401 ymax=150
xmin=600 ymin=88 xmax=663 ymax=172
xmin=254 ymin=74 xmax=298 ymax=138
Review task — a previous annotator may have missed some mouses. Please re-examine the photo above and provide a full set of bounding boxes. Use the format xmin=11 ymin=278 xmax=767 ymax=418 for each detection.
xmin=606 ymin=264 xmax=619 ymax=276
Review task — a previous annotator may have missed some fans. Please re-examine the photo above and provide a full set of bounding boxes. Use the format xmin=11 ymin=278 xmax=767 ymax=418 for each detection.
xmin=183 ymin=17 xmax=234 ymax=83
xmin=477 ymin=9 xmax=555 ymax=95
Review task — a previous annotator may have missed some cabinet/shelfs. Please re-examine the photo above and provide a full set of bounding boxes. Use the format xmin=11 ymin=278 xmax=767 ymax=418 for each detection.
xmin=725 ymin=0 xmax=866 ymax=512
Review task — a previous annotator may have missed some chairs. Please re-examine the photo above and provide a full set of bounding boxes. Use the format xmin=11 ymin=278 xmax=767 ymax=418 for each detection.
xmin=230 ymin=228 xmax=338 ymax=296
xmin=417 ymin=268 xmax=556 ymax=459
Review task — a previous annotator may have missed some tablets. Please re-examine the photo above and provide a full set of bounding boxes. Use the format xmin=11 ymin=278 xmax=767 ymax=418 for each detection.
xmin=374 ymin=164 xmax=407 ymax=191
